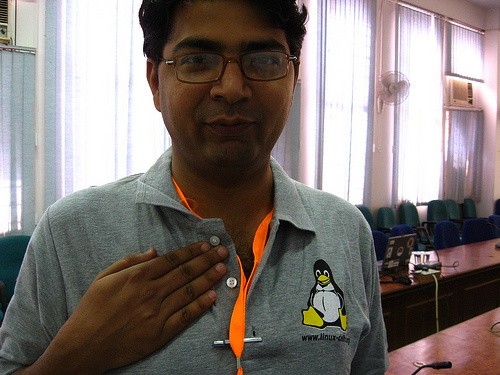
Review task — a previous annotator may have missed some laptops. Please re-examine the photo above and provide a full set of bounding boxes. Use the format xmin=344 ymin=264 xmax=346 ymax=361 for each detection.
xmin=377 ymin=233 xmax=416 ymax=274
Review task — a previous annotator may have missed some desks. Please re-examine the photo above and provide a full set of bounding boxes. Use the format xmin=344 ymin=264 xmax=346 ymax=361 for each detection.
xmin=376 ymin=238 xmax=500 ymax=352
xmin=385 ymin=306 xmax=500 ymax=375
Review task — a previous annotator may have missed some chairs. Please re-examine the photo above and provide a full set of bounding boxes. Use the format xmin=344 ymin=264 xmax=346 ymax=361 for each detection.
xmin=355 ymin=198 xmax=500 ymax=261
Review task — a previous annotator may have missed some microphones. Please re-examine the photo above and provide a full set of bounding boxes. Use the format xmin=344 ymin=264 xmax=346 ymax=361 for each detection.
xmin=412 ymin=361 xmax=452 ymax=375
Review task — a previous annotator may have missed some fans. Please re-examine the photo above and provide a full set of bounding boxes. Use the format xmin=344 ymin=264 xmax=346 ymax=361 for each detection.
xmin=376 ymin=70 xmax=411 ymax=114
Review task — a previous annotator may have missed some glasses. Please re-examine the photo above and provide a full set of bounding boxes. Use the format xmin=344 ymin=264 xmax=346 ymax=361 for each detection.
xmin=155 ymin=48 xmax=297 ymax=84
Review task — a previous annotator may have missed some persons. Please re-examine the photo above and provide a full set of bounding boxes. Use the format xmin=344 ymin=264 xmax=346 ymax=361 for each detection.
xmin=0 ymin=0 xmax=390 ymax=375
